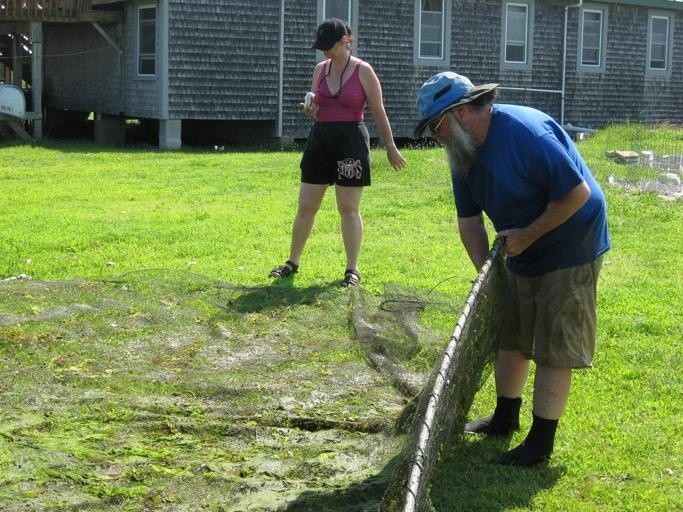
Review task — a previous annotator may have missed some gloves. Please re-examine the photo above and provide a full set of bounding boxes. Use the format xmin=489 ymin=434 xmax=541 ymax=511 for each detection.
xmin=304 ymin=92 xmax=320 ymax=112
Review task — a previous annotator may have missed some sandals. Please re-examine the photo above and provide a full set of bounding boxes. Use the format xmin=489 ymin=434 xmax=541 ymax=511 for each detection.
xmin=341 ymin=269 xmax=360 ymax=288
xmin=270 ymin=260 xmax=299 ymax=278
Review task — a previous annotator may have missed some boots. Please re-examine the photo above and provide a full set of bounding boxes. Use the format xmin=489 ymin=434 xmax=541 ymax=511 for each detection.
xmin=464 ymin=396 xmax=559 ymax=467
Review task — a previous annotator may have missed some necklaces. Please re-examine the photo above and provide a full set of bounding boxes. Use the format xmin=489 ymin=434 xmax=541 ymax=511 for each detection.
xmin=313 ymin=49 xmax=355 ymax=103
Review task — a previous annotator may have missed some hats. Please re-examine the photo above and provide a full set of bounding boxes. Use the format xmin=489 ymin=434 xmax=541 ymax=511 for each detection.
xmin=413 ymin=71 xmax=500 ymax=139
xmin=312 ymin=17 xmax=349 ymax=51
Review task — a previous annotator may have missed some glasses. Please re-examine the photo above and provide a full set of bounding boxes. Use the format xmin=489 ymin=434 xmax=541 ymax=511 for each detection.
xmin=429 ymin=113 xmax=448 ymax=139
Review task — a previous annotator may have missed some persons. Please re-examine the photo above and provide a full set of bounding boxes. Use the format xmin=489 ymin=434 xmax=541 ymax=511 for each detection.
xmin=265 ymin=16 xmax=406 ymax=286
xmin=411 ymin=72 xmax=611 ymax=467
